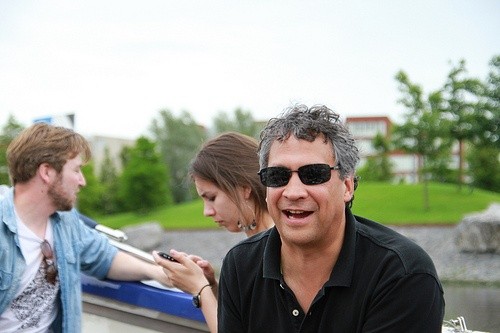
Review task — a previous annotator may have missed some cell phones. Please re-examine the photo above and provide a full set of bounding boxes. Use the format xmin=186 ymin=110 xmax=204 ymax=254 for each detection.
xmin=156 ymin=251 xmax=179 ymax=263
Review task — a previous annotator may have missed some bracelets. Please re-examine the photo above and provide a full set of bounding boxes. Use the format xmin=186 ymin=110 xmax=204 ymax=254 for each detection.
xmin=192 ymin=283 xmax=213 ymax=308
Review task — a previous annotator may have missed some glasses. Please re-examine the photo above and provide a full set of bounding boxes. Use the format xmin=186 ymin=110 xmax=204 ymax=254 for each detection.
xmin=40 ymin=239 xmax=58 ymax=285
xmin=258 ymin=164 xmax=341 ymax=188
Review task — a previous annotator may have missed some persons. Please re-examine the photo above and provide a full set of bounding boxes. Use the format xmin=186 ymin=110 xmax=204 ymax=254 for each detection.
xmin=217 ymin=105 xmax=445 ymax=333
xmin=0 ymin=122 xmax=174 ymax=333
xmin=152 ymin=132 xmax=275 ymax=333
